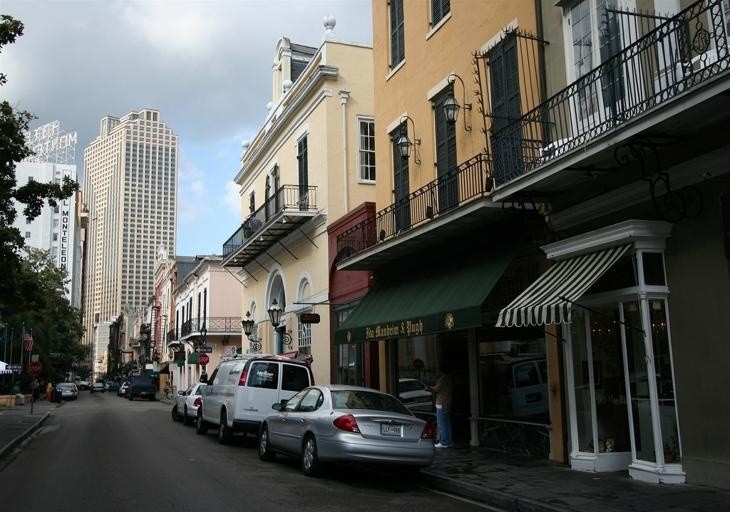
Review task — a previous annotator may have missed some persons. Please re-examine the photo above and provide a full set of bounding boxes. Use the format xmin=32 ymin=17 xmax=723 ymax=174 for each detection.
xmin=424 ymin=358 xmax=461 ymax=449
xmin=413 ymin=358 xmax=437 ymax=383
xmin=12 ymin=381 xmax=26 ymax=406
xmin=31 ymin=379 xmax=40 ymax=402
xmin=46 ymin=379 xmax=53 ymax=401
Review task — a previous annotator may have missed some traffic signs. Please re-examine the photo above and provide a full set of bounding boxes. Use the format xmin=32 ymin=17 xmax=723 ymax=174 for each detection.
xmin=5 ymin=365 xmax=24 ymax=370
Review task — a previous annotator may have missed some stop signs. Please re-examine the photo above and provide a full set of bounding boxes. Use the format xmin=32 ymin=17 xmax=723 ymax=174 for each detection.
xmin=198 ymin=354 xmax=209 ymax=365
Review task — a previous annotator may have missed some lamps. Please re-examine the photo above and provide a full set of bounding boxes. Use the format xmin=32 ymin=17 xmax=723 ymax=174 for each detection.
xmin=397 ymin=74 xmax=472 ymax=166
xmin=242 ymin=296 xmax=294 ymax=354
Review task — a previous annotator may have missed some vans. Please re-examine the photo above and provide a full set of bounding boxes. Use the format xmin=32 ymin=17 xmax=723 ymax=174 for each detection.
xmin=197 ymin=353 xmax=315 ymax=444
xmin=481 ymin=351 xmax=548 ymax=420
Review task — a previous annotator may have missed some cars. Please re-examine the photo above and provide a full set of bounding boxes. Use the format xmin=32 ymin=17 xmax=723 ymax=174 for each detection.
xmin=399 ymin=377 xmax=434 ymax=413
xmin=56 ymin=376 xmax=157 ymax=400
xmin=259 ymin=384 xmax=435 ymax=476
xmin=172 ymin=383 xmax=207 ymax=426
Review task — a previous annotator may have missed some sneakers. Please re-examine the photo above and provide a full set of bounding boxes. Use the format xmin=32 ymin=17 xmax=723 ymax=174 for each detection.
xmin=434 ymin=442 xmax=453 ymax=448
